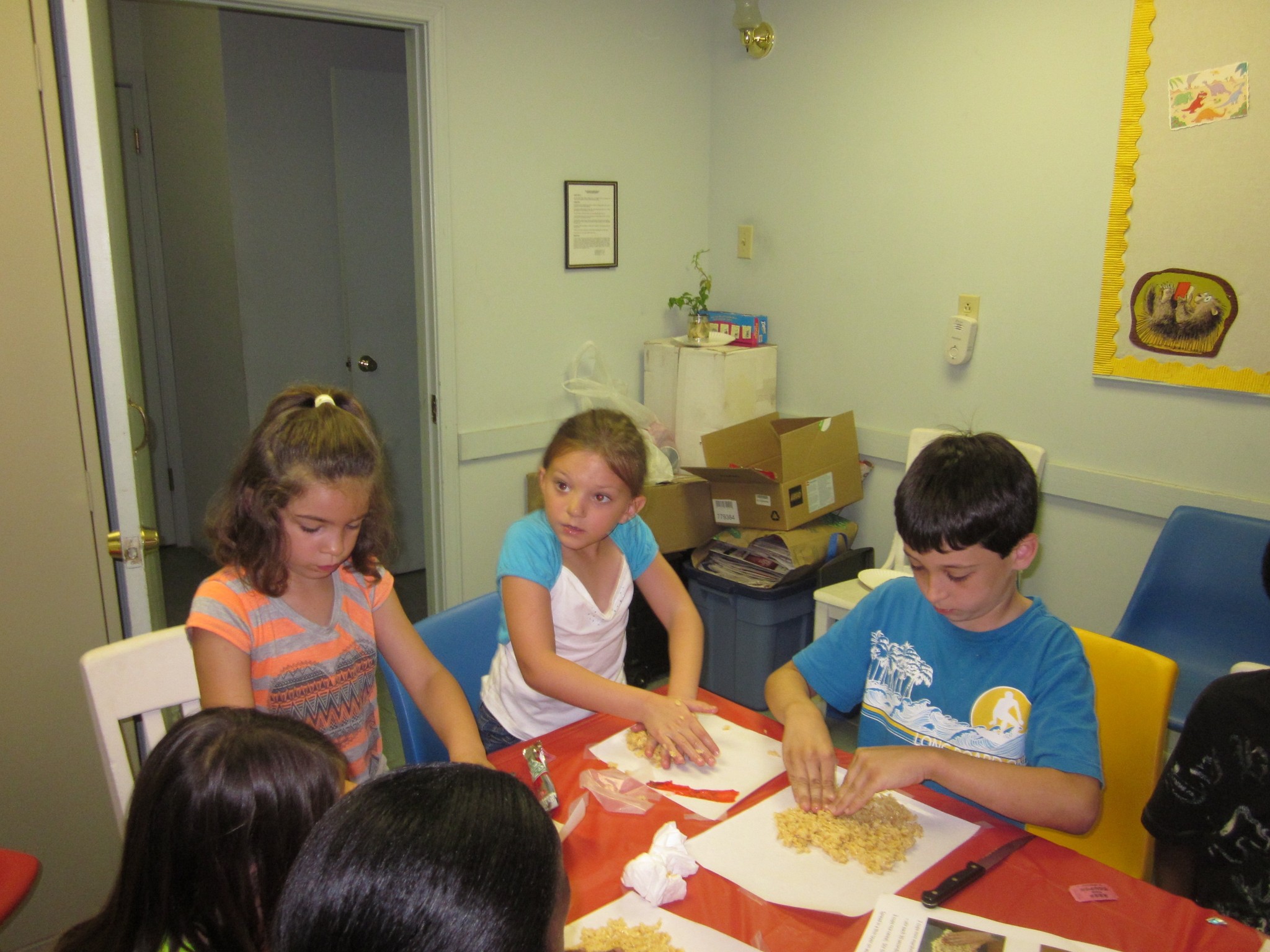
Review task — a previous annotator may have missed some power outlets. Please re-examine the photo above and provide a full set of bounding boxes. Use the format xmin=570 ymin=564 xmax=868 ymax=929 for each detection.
xmin=958 ymin=294 xmax=980 ymax=320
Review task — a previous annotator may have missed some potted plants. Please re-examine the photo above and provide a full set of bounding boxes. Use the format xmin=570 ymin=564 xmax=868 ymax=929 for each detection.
xmin=669 ymin=248 xmax=711 ymax=342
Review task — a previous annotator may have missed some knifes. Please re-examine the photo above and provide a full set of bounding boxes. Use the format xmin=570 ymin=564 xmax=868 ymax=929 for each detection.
xmin=920 ymin=834 xmax=1037 ymax=908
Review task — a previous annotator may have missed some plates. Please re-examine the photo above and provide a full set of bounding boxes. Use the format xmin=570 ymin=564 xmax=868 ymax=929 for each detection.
xmin=672 ymin=332 xmax=737 ymax=348
xmin=858 ymin=569 xmax=914 ymax=590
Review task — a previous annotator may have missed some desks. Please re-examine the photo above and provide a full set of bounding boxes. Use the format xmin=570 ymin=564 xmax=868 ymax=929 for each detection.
xmin=484 ymin=686 xmax=1269 ymax=952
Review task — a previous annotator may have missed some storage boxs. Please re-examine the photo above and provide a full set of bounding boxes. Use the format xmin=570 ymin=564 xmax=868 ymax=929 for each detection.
xmin=527 ymin=469 xmax=717 ymax=553
xmin=698 ymin=311 xmax=768 ymax=343
xmin=680 ymin=411 xmax=864 ymax=531
xmin=688 ymin=579 xmax=815 ymax=710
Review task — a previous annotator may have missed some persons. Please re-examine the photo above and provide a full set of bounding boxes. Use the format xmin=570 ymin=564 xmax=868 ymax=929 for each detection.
xmin=766 ymin=432 xmax=1103 ymax=836
xmin=184 ymin=386 xmax=495 ymax=797
xmin=477 ymin=407 xmax=719 ymax=772
xmin=48 ymin=704 xmax=570 ymax=952
xmin=940 ymin=929 xmax=1002 ymax=952
xmin=1141 ymin=539 xmax=1270 ymax=941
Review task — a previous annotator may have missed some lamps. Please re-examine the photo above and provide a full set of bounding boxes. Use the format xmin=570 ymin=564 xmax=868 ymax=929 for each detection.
xmin=733 ymin=0 xmax=774 ymax=59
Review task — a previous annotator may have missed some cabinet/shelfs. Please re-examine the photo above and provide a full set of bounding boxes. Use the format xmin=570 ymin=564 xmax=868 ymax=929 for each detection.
xmin=644 ymin=337 xmax=777 ymax=475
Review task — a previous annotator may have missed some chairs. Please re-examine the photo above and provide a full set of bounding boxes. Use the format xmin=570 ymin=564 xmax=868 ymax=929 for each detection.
xmin=813 ymin=426 xmax=1047 ymax=644
xmin=78 ymin=623 xmax=202 ymax=850
xmin=1109 ymin=506 xmax=1269 ymax=732
xmin=1024 ymin=627 xmax=1178 ymax=886
xmin=374 ymin=591 xmax=501 ymax=767
